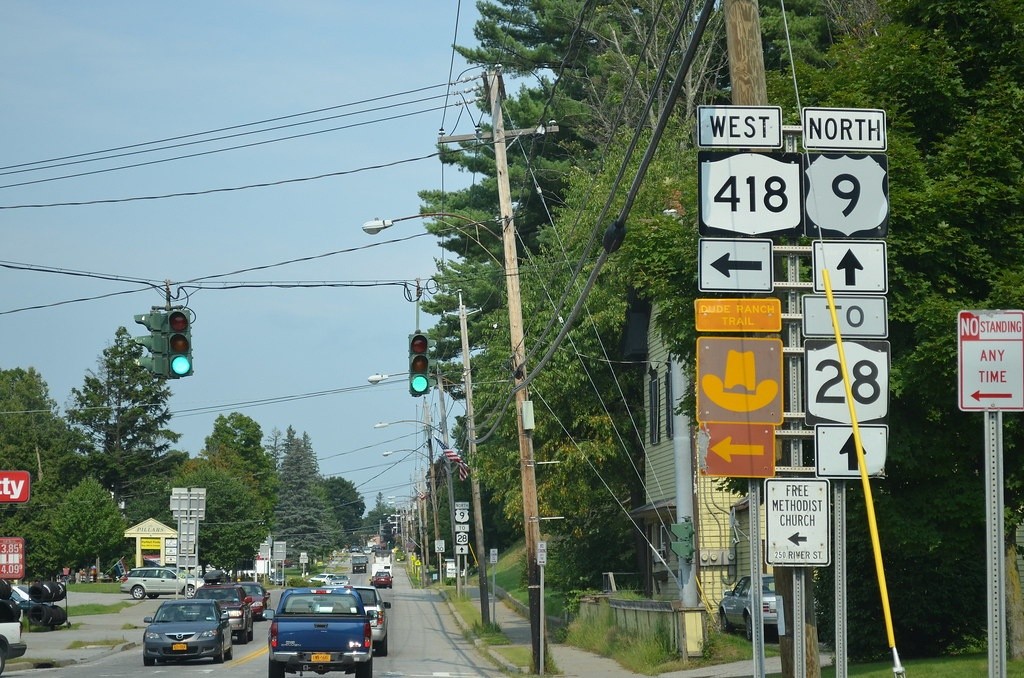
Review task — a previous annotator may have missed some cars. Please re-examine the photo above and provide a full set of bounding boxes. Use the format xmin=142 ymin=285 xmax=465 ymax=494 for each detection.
xmin=11 ymin=586 xmax=52 ymax=607
xmin=718 ymin=574 xmax=779 ymax=642
xmin=142 ymin=598 xmax=235 ymax=667
xmin=351 ymin=544 xmax=394 ymax=590
xmin=233 ymin=582 xmax=271 ymax=622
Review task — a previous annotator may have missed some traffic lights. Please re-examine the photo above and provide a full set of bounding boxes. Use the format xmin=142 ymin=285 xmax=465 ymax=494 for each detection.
xmin=408 ymin=333 xmax=430 ymax=397
xmin=133 ymin=313 xmax=166 ymax=378
xmin=166 ymin=310 xmax=194 ymax=378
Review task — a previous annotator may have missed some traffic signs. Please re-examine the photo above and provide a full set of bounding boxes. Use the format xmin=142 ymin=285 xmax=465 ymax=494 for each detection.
xmin=764 ymin=476 xmax=831 ymax=567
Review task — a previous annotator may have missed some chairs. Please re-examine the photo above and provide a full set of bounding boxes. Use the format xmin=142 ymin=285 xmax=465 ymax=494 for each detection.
xmin=199 ymin=606 xmax=213 ymax=620
xmin=289 ymin=599 xmax=310 ymax=613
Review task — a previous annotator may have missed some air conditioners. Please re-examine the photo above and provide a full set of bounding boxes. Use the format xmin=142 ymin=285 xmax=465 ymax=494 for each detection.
xmin=654 ymin=549 xmax=667 ymax=563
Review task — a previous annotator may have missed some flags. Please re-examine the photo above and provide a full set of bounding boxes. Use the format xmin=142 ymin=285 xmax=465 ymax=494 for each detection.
xmin=412 ymin=487 xmax=427 ymax=501
xmin=405 ymin=537 xmax=416 ymax=550
xmin=433 ymin=436 xmax=468 ymax=481
xmin=115 ymin=560 xmax=129 ymax=576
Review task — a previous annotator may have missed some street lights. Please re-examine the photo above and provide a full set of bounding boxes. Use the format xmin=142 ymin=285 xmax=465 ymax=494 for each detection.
xmin=362 ymin=211 xmax=547 ymax=667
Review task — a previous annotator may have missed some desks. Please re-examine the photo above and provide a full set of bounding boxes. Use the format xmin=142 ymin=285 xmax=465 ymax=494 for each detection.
xmin=98 ymin=577 xmax=114 ymax=583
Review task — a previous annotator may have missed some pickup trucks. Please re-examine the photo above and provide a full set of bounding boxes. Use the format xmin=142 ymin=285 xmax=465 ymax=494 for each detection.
xmin=267 ymin=586 xmax=374 ymax=678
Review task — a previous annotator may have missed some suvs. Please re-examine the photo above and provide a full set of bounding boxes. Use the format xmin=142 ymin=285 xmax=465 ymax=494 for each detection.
xmin=119 ymin=567 xmax=205 ymax=599
xmin=307 ymin=586 xmax=391 ymax=657
xmin=191 ymin=585 xmax=254 ymax=645
